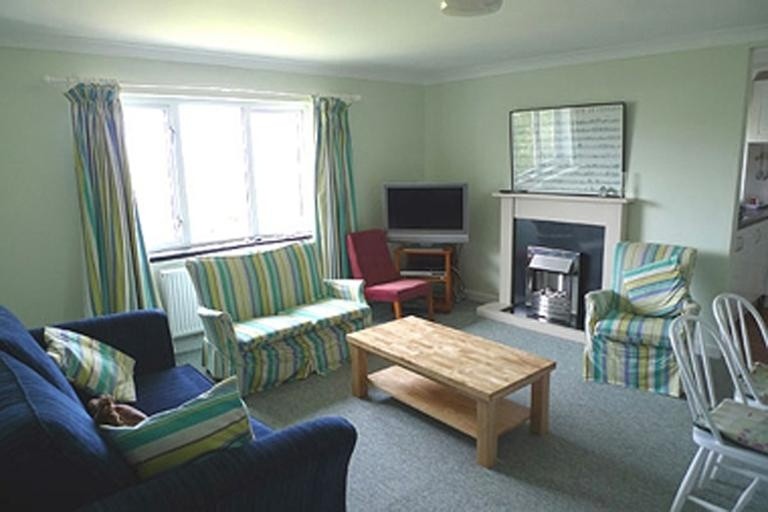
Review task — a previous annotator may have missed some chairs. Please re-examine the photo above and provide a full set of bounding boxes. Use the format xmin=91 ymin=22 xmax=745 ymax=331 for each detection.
xmin=712 ymin=292 xmax=768 ymax=405
xmin=346 ymin=230 xmax=433 ymax=319
xmin=670 ymin=315 xmax=768 ymax=512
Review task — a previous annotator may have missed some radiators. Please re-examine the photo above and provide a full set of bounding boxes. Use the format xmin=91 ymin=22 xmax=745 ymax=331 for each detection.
xmin=158 ymin=267 xmax=204 ymax=339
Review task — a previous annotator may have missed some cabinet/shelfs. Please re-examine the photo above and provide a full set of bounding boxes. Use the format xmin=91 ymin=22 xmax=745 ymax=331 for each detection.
xmin=392 ymin=246 xmax=455 ymax=313
xmin=726 ymin=206 xmax=768 ymax=324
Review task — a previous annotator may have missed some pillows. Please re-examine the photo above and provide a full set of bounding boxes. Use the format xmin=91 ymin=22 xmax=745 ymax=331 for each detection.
xmin=99 ymin=376 xmax=254 ymax=480
xmin=622 ymin=256 xmax=691 ymax=316
xmin=45 ymin=326 xmax=137 ymax=404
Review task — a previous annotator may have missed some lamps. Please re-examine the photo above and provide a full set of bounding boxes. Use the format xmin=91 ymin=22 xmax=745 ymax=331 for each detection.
xmin=440 ymin=0 xmax=503 ymax=18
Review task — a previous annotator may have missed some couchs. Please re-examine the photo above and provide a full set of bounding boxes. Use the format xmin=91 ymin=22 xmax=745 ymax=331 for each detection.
xmin=0 ymin=306 xmax=358 ymax=511
xmin=582 ymin=240 xmax=700 ymax=399
xmin=186 ymin=239 xmax=371 ymax=397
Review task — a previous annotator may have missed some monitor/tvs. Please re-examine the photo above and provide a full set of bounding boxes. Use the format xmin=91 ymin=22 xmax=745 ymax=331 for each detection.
xmin=381 ymin=181 xmax=473 ymax=246
